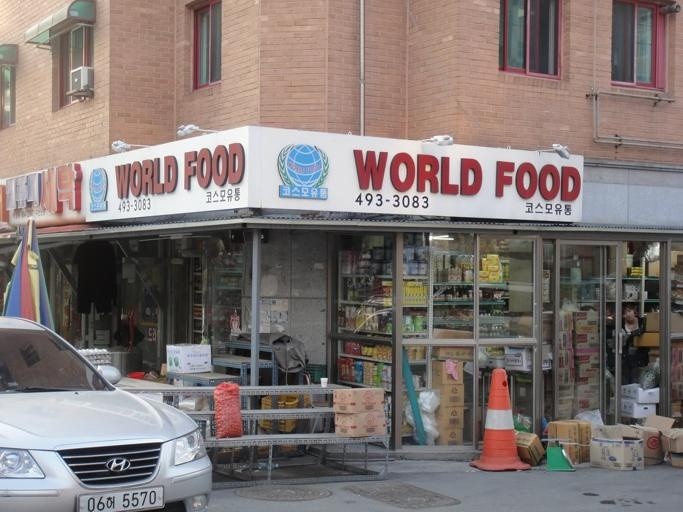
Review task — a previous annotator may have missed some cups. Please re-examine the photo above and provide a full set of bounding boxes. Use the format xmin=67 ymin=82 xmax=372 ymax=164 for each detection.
xmin=320 ymin=377 xmax=328 ymax=388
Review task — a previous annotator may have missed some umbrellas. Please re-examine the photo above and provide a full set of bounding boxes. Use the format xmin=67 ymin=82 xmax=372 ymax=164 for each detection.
xmin=2 ymin=217 xmax=55 ymax=333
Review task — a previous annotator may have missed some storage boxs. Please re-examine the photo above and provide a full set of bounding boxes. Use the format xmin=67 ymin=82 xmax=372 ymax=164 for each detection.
xmin=514 ymin=416 xmax=683 ymax=470
xmin=166 ymin=344 xmax=212 ymax=375
xmin=427 ymin=357 xmax=464 ymax=446
xmin=332 ymin=389 xmax=384 ymax=437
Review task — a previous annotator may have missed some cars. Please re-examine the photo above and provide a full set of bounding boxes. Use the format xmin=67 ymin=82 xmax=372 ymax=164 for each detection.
xmin=1 ymin=315 xmax=213 ymax=512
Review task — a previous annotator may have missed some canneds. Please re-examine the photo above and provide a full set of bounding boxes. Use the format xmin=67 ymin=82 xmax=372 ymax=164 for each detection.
xmin=338 ymin=233 xmax=430 ymax=275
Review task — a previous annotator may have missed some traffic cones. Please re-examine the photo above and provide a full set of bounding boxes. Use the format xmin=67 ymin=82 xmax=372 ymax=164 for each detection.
xmin=466 ymin=366 xmax=533 ymax=473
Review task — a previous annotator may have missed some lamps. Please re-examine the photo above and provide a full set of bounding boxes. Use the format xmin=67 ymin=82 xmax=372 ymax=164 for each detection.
xmin=536 ymin=142 xmax=571 ymax=160
xmin=419 ymin=133 xmax=455 ymax=146
xmin=111 ymin=139 xmax=154 ymax=153
xmin=173 ymin=121 xmax=220 ymax=138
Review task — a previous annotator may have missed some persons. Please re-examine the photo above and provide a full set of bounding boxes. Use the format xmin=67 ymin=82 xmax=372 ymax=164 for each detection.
xmin=616 ymin=301 xmax=651 ymax=366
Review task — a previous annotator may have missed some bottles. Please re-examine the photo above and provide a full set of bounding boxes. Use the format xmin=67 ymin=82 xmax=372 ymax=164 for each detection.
xmin=480 ymin=310 xmax=506 ymax=337
xmin=386 ymin=315 xmax=424 ymax=333
